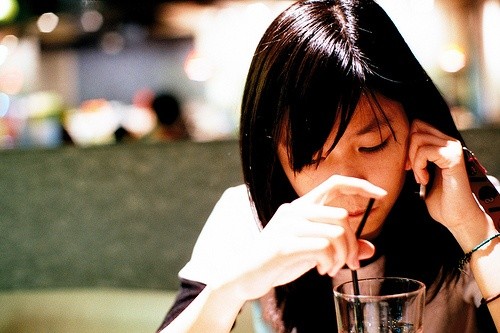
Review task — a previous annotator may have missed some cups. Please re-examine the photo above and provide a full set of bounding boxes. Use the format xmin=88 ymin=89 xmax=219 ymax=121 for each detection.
xmin=333 ymin=276 xmax=428 ymax=333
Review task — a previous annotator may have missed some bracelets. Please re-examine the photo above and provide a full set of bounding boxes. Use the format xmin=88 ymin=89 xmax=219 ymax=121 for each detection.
xmin=464 ymin=232 xmax=500 ymax=258
xmin=483 ymin=293 xmax=499 ymax=303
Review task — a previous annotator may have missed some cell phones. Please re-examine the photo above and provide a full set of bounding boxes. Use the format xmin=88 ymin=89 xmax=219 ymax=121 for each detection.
xmin=410 ymin=162 xmax=434 ymax=199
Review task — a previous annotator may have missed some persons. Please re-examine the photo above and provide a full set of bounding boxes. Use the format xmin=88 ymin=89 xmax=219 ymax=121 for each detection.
xmin=155 ymin=1 xmax=500 ymax=333
